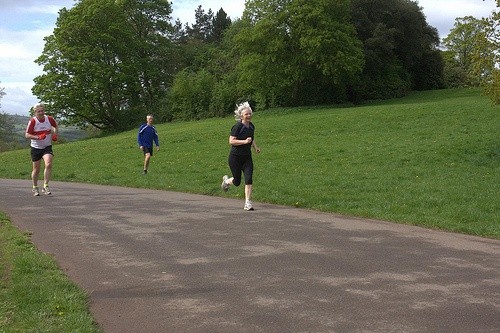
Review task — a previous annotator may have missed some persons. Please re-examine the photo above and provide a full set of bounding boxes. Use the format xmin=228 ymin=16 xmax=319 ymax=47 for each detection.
xmin=137 ymin=113 xmax=160 ymax=174
xmin=25 ymin=103 xmax=60 ymax=196
xmin=221 ymin=102 xmax=261 ymax=211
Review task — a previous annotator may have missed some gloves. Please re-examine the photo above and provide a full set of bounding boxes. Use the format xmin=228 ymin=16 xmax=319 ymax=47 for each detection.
xmin=51 ymin=134 xmax=58 ymax=142
xmin=38 ymin=134 xmax=46 ymax=141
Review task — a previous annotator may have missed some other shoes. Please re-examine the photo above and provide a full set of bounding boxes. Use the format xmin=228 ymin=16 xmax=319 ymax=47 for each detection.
xmin=143 ymin=170 xmax=146 ymax=174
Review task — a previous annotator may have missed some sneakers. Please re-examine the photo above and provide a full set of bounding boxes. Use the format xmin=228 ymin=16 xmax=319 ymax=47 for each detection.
xmin=32 ymin=188 xmax=39 ymax=197
xmin=244 ymin=200 xmax=254 ymax=210
xmin=221 ymin=175 xmax=229 ymax=192
xmin=42 ymin=187 xmax=52 ymax=196
